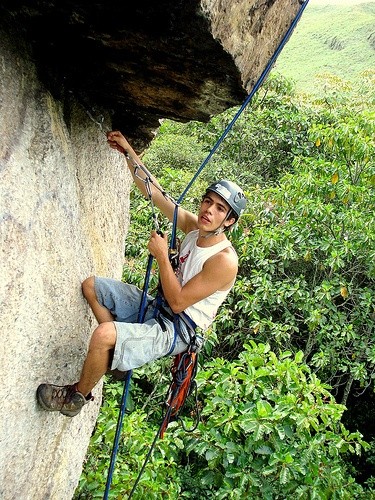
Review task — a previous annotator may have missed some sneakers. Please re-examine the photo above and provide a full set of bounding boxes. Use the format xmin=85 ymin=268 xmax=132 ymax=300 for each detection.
xmin=36 ymin=382 xmax=94 ymax=418
xmin=107 ymin=368 xmax=130 ymax=381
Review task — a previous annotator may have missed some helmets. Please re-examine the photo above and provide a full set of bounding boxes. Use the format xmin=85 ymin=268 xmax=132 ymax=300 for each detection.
xmin=205 ymin=179 xmax=248 ymax=215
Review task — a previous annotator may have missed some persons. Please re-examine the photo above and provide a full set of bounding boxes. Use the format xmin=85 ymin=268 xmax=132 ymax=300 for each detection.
xmin=37 ymin=131 xmax=246 ymax=418
